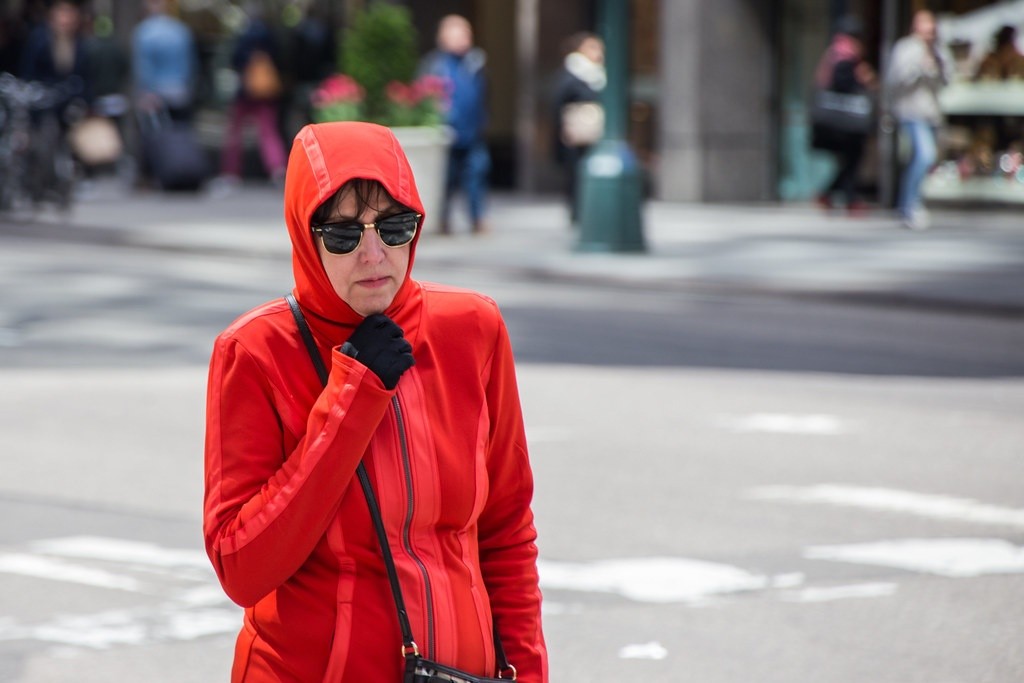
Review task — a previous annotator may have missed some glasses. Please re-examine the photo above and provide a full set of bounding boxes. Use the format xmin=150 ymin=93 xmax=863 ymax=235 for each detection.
xmin=310 ymin=210 xmax=423 ymax=256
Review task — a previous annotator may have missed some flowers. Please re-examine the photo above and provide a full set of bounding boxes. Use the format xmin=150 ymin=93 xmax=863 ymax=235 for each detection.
xmin=328 ymin=3 xmax=455 ymax=124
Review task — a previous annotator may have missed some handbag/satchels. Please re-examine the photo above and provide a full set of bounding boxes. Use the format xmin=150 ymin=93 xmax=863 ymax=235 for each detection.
xmin=563 ymin=103 xmax=603 ymax=145
xmin=811 ymin=93 xmax=876 ymax=135
xmin=402 ymin=651 xmax=517 ymax=683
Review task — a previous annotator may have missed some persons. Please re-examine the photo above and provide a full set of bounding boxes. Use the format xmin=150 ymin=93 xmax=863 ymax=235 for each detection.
xmin=19 ymin=0 xmax=337 ymax=193
xmin=548 ymin=30 xmax=609 ymax=220
xmin=415 ymin=15 xmax=492 ymax=236
xmin=813 ymin=13 xmax=879 ymax=213
xmin=974 ymin=27 xmax=1024 ymax=82
xmin=887 ymin=8 xmax=945 ymax=227
xmin=202 ymin=120 xmax=549 ymax=683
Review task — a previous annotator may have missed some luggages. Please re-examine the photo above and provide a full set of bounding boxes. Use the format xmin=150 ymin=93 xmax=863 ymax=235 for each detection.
xmin=132 ymin=103 xmax=210 ymax=193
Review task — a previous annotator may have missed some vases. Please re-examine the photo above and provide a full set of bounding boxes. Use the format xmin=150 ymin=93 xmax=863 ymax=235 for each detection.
xmin=383 ymin=123 xmax=459 ymax=237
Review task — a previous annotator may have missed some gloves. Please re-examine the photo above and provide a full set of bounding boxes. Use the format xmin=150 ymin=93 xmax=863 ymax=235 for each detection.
xmin=339 ymin=312 xmax=416 ymax=389
